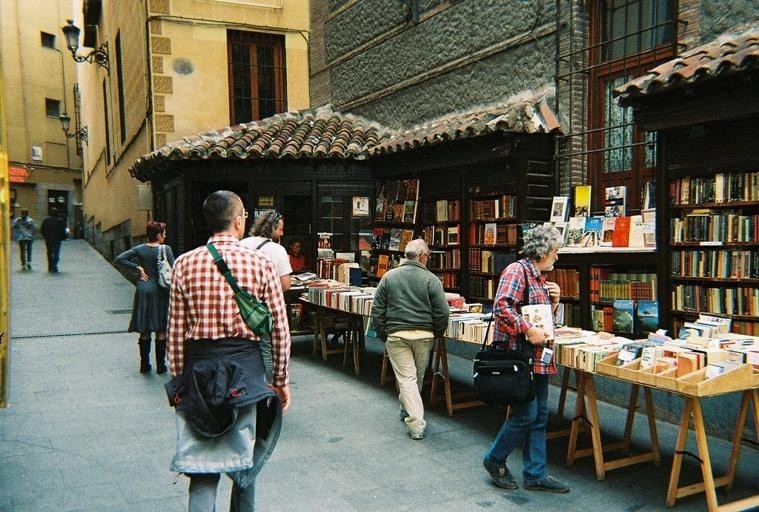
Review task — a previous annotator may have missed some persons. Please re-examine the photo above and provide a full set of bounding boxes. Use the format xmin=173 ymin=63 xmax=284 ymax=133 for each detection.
xmin=112 ymin=219 xmax=175 ymax=373
xmin=41 ymin=206 xmax=66 ymax=273
xmin=165 ymin=189 xmax=291 ymax=512
xmin=370 ymin=239 xmax=450 ymax=441
xmin=287 ymin=240 xmax=305 ymax=270
xmin=238 ymin=210 xmax=291 ymax=292
xmin=11 ymin=208 xmax=38 ymax=270
xmin=482 ymin=223 xmax=571 ymax=492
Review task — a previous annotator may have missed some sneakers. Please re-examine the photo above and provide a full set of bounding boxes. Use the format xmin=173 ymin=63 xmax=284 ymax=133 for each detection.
xmin=483 ymin=457 xmax=519 ymax=490
xmin=406 ymin=426 xmax=423 ymax=440
xmin=399 ymin=411 xmax=409 ymax=420
xmin=524 ymin=475 xmax=570 ymax=493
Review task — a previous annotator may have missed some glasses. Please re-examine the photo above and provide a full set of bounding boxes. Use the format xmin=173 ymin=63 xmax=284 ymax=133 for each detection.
xmin=241 ymin=212 xmax=248 ymax=219
xmin=427 ymin=255 xmax=432 ymax=261
xmin=275 ymin=213 xmax=280 ymax=220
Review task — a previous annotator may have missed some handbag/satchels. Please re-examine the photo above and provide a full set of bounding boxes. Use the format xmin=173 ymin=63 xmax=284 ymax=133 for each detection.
xmin=473 ymin=340 xmax=536 ymax=404
xmin=157 ymin=245 xmax=172 ymax=290
xmin=235 ymin=289 xmax=273 ymax=336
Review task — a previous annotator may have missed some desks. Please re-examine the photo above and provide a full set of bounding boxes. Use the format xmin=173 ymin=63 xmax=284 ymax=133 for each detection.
xmin=566 ymin=363 xmax=758 ymax=511
xmin=312 ymin=303 xmax=442 ymax=384
xmin=282 ymin=286 xmax=366 ymax=354
xmin=430 ymin=334 xmax=583 ymax=440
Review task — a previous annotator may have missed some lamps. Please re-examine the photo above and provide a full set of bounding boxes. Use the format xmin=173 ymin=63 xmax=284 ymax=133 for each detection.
xmin=61 ymin=19 xmax=110 ymax=75
xmin=59 ymin=111 xmax=88 ymax=147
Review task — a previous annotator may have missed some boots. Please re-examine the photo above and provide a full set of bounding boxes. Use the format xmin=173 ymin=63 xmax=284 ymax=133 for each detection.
xmin=139 ymin=338 xmax=152 ymax=373
xmin=155 ymin=339 xmax=167 ymax=374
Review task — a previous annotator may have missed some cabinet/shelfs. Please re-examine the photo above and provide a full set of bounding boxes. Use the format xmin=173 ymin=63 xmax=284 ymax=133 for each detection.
xmin=366 ymin=155 xmax=556 ymax=305
xmin=657 ymin=159 xmax=759 ymax=340
xmin=545 ymin=246 xmax=659 ymax=339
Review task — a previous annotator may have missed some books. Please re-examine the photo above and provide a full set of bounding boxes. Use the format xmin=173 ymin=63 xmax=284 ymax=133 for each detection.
xmin=616 ymin=314 xmax=759 ymax=381
xmin=670 ymin=284 xmax=758 ymax=316
xmin=469 ymin=274 xmax=496 ymax=300
xmin=471 ymin=194 xmax=519 ymax=220
xmin=427 ymin=248 xmax=461 ymax=270
xmin=551 ymin=325 xmax=635 ymax=373
xmin=309 ymin=279 xmax=377 ymax=317
xmin=374 ymin=179 xmax=419 ymax=223
xmin=665 ymin=171 xmax=759 ymax=204
xmin=667 ymin=208 xmax=759 ymax=242
xmin=537 ymin=266 xmax=581 ymax=299
xmin=469 ymin=222 xmax=517 ymax=247
xmin=549 ymin=183 xmax=656 ymax=246
xmin=422 ymin=199 xmax=461 ymax=221
xmin=591 ymin=267 xmax=660 ymax=303
xmin=671 ymin=248 xmax=758 ymax=279
xmin=520 ymin=304 xmax=555 ymax=341
xmin=318 ymin=232 xmax=361 ymax=286
xmin=423 ymin=226 xmax=461 ymax=246
xmin=431 ymin=272 xmax=461 ymax=289
xmin=291 ymin=271 xmax=317 ymax=301
xmin=469 ymin=249 xmax=517 ymax=274
xmin=439 ymin=293 xmax=495 ymax=346
xmin=589 ymin=301 xmax=661 ymax=338
